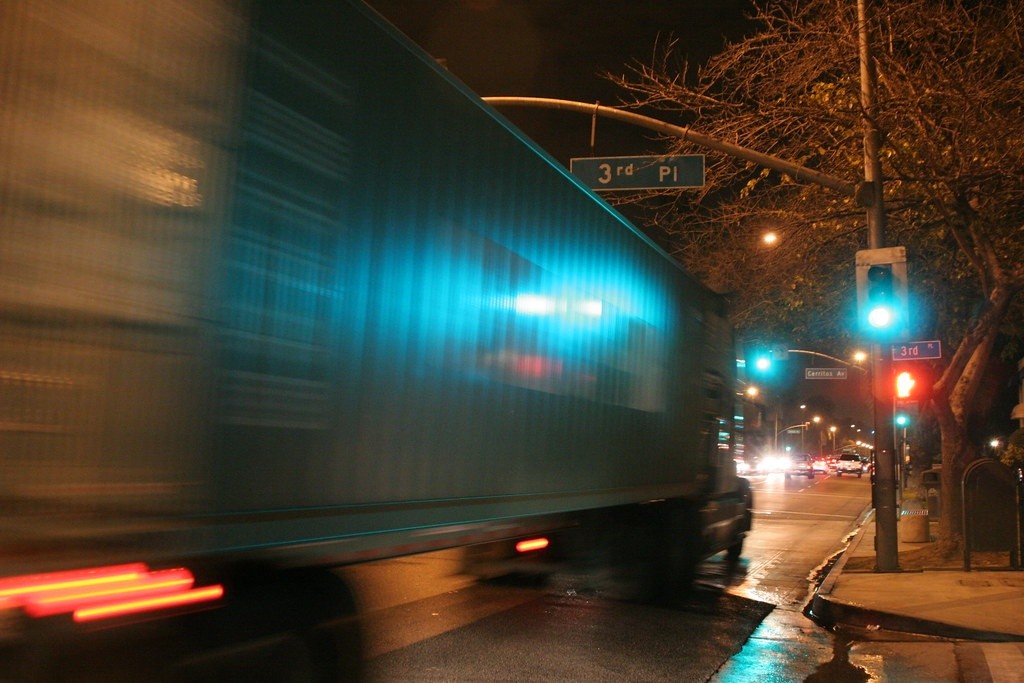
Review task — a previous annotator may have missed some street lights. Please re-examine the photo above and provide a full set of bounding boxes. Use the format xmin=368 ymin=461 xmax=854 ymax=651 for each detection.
xmin=774 ymin=421 xmax=810 ymax=457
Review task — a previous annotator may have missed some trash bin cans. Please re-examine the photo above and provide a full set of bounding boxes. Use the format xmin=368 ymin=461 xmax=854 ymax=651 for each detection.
xmin=919 ymin=469 xmax=942 ymax=521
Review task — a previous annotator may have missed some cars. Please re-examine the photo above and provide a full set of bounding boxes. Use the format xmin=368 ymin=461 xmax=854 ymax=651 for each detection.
xmin=733 ymin=450 xmax=872 ymax=482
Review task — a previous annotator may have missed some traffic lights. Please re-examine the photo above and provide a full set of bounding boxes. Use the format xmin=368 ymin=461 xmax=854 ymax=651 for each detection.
xmin=894 ymin=408 xmax=912 ymax=429
xmin=745 ymin=339 xmax=776 ymax=382
xmin=851 ymin=246 xmax=911 ymax=344
xmin=894 ymin=363 xmax=922 ymax=406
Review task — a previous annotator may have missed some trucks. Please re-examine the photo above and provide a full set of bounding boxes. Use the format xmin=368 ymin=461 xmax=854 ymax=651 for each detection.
xmin=0 ymin=1 xmax=754 ymax=683
